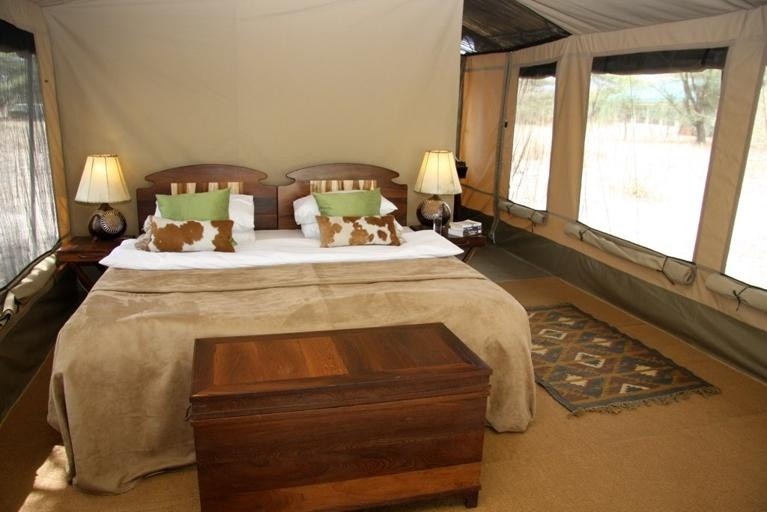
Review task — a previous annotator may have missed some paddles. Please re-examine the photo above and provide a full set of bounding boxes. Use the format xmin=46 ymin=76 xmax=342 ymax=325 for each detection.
xmin=523 ymin=301 xmax=721 ymax=419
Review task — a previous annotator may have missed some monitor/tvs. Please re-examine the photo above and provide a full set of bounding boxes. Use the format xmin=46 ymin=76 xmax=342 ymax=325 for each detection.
xmin=446 ymin=219 xmax=482 ymax=239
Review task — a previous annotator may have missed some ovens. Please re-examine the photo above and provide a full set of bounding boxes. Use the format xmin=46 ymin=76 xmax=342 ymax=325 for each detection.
xmin=61 ymin=163 xmax=519 ymax=478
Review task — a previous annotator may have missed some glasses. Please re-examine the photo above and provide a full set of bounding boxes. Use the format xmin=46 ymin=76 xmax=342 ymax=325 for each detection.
xmin=414 ymin=149 xmax=463 ymax=227
xmin=74 ymin=153 xmax=132 ymax=242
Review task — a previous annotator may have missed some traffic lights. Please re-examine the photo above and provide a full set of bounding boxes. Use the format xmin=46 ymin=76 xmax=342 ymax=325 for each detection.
xmin=135 ymin=186 xmax=256 ymax=254
xmin=292 ymin=188 xmax=407 ymax=248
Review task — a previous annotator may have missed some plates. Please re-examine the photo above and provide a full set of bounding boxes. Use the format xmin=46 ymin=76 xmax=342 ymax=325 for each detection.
xmin=188 ymin=322 xmax=493 ymax=512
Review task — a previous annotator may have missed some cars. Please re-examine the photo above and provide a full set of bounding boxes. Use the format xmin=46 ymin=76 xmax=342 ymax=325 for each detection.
xmin=431 ymin=213 xmax=443 ymax=236
